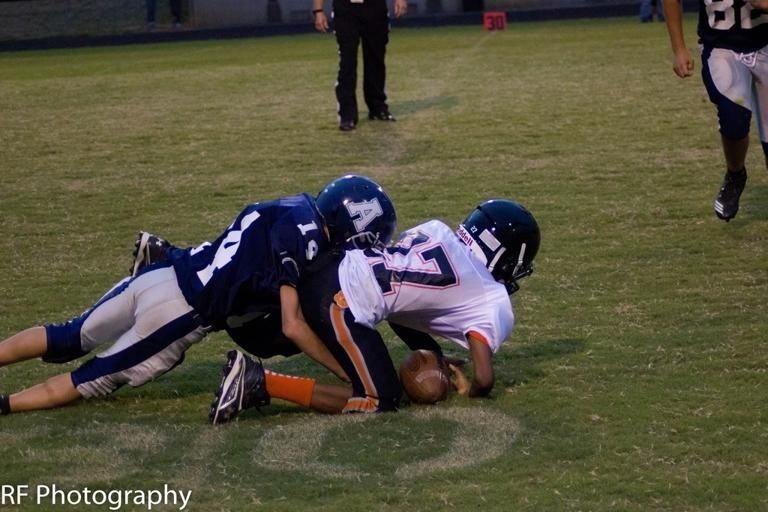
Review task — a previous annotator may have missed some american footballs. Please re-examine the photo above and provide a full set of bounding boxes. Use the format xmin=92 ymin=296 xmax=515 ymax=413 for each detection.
xmin=400 ymin=349 xmax=450 ymax=402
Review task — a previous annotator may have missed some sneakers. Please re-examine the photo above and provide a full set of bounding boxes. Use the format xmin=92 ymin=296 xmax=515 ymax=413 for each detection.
xmin=367 ymin=109 xmax=397 ymax=121
xmin=337 ymin=115 xmax=359 ymax=130
xmin=206 ymin=347 xmax=274 ymax=427
xmin=712 ymin=167 xmax=749 ymax=224
xmin=127 ymin=230 xmax=171 ymax=277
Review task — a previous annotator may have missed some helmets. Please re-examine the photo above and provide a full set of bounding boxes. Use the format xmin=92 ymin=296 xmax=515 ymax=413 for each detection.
xmin=455 ymin=197 xmax=542 ymax=297
xmin=312 ymin=174 xmax=398 ymax=261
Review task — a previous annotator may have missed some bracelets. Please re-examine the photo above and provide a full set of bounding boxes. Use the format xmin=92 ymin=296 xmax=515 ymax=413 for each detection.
xmin=313 ymin=9 xmax=322 ymax=12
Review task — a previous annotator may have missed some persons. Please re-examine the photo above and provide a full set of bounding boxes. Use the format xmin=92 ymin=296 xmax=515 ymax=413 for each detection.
xmin=0 ymin=175 xmax=396 ymax=416
xmin=128 ymin=199 xmax=540 ymax=426
xmin=662 ymin=0 xmax=768 ymax=221
xmin=313 ymin=0 xmax=408 ymax=130
